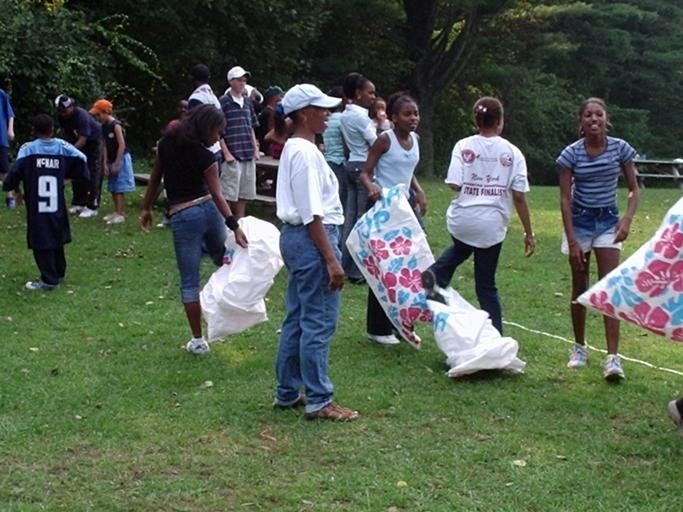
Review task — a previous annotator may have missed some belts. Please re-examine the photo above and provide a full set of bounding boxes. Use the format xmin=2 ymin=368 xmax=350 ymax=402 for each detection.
xmin=169 ymin=194 xmax=212 ymax=218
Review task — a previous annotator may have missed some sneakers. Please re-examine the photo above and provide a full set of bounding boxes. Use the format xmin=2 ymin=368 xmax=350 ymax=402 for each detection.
xmin=667 ymin=397 xmax=683 ymax=430
xmin=567 ymin=342 xmax=591 ymax=371
xmin=421 ymin=268 xmax=438 ymax=293
xmin=69 ymin=204 xmax=85 ymax=215
xmin=25 ymin=278 xmax=60 ymax=293
xmin=275 ymin=395 xmax=310 ymax=409
xmin=604 ymin=353 xmax=625 ymax=382
xmin=79 ymin=204 xmax=99 ymax=219
xmin=103 ymin=210 xmax=120 ymax=222
xmin=309 ymin=403 xmax=360 ymax=421
xmin=185 ymin=337 xmax=212 ymax=356
xmin=107 ymin=216 xmax=126 ymax=226
xmin=155 ymin=213 xmax=171 ymax=230
xmin=367 ymin=332 xmax=400 ymax=345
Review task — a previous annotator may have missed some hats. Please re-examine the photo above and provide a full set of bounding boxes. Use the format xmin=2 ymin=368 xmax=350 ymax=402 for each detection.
xmin=265 ymin=85 xmax=286 ymax=98
xmin=280 ymin=84 xmax=342 ymax=114
xmin=88 ymin=98 xmax=114 ymax=114
xmin=227 ymin=66 xmax=250 ymax=82
xmin=53 ymin=94 xmax=75 ymax=108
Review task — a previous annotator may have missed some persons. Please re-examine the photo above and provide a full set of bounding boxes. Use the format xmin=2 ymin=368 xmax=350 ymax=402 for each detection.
xmin=189 ymin=64 xmax=224 ymax=177
xmin=138 ymin=104 xmax=247 ymax=357
xmin=340 ymin=73 xmax=378 ymax=284
xmin=219 ymin=66 xmax=260 ymax=220
xmin=2 ymin=114 xmax=88 ymax=291
xmin=359 ymin=93 xmax=428 ymax=345
xmin=165 ymin=99 xmax=188 ymax=136
xmin=370 ymin=97 xmax=394 ymax=136
xmin=322 ymin=86 xmax=348 ymax=208
xmin=556 ymin=97 xmax=640 ymax=383
xmin=0 ymin=86 xmax=15 ymax=208
xmin=89 ymin=100 xmax=136 ymax=223
xmin=270 ymin=85 xmax=360 ymax=423
xmin=420 ymin=97 xmax=535 ymax=338
xmin=225 ymin=85 xmax=292 ymax=159
xmin=54 ymin=94 xmax=103 ymax=218
xmin=668 ymin=397 xmax=683 ymax=425
